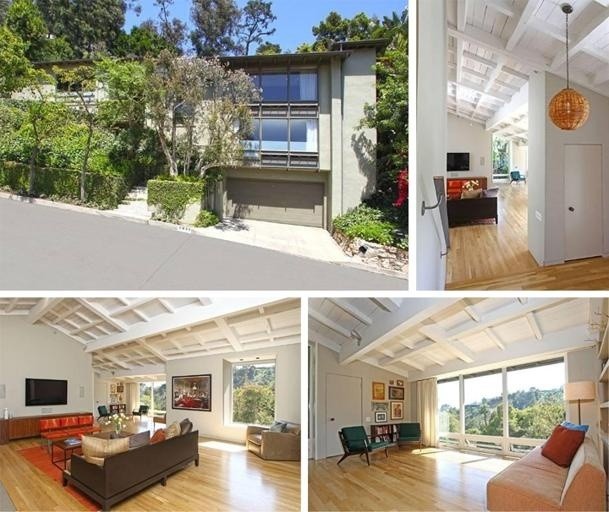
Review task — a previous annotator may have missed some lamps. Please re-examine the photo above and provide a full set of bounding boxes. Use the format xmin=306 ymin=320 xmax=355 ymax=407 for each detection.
xmin=549 ymin=5 xmax=589 ymax=130
xmin=564 ymin=382 xmax=595 ymax=424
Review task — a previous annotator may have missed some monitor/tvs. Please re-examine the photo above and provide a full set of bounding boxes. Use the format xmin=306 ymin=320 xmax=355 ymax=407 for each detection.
xmin=447 ymin=152 xmax=470 ymax=170
xmin=25 ymin=377 xmax=68 ymax=407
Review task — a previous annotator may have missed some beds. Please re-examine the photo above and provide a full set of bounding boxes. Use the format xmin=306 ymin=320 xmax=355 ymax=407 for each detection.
xmin=50 ymin=438 xmax=82 ymax=469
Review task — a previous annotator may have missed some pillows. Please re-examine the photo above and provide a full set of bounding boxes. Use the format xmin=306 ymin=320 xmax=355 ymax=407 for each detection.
xmin=482 ymin=187 xmax=500 ymax=197
xmin=78 ymin=419 xmax=193 ymax=468
xmin=542 ymin=424 xmax=585 ymax=467
xmin=461 ymin=188 xmax=482 ymax=200
xmin=270 ymin=422 xmax=287 ymax=432
xmin=561 ymin=421 xmax=590 ymax=432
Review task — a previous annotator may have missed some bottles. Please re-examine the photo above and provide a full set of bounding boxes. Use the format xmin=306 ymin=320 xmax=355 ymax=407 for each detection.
xmin=3 ymin=408 xmax=8 ymax=420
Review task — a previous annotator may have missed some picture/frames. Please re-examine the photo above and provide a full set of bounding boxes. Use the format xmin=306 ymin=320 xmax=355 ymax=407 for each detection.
xmin=110 ymin=382 xmax=124 ymax=402
xmin=372 ymin=379 xmax=404 ymax=422
xmin=171 ymin=373 xmax=211 ymax=412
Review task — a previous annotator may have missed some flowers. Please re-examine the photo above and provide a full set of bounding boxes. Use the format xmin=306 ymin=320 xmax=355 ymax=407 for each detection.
xmin=462 ymin=180 xmax=480 ymax=190
xmin=104 ymin=413 xmax=129 ymax=426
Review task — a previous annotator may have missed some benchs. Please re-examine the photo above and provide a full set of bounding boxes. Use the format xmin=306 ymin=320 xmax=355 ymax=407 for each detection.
xmin=447 ymin=197 xmax=498 ymax=226
xmin=39 ymin=415 xmax=101 ymax=453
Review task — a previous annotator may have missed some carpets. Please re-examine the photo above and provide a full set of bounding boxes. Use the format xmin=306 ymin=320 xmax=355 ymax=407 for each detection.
xmin=17 ymin=445 xmax=104 ymax=512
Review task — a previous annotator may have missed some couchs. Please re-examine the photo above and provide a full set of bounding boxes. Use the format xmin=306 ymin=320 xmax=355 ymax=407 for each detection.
xmin=246 ymin=419 xmax=301 ymax=462
xmin=63 ymin=419 xmax=199 ymax=512
xmin=486 ymin=435 xmax=606 ymax=512
xmin=132 ymin=405 xmax=149 ymax=421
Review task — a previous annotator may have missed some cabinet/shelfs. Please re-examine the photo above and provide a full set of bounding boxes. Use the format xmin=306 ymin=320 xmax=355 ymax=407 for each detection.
xmin=0 ymin=416 xmax=39 ymax=444
xmin=370 ymin=424 xmax=396 ymax=444
xmin=447 ymin=176 xmax=487 ymax=194
xmin=110 ymin=404 xmax=126 ymax=415
xmin=597 ymin=304 xmax=609 ymax=475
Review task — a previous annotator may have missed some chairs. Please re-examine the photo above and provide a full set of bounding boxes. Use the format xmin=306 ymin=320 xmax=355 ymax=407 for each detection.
xmin=510 ymin=171 xmax=527 ymax=186
xmin=97 ymin=405 xmax=111 ymax=421
xmin=337 ymin=427 xmax=390 ymax=465
xmin=153 ymin=413 xmax=166 ymax=427
xmin=396 ymin=423 xmax=421 ymax=450
